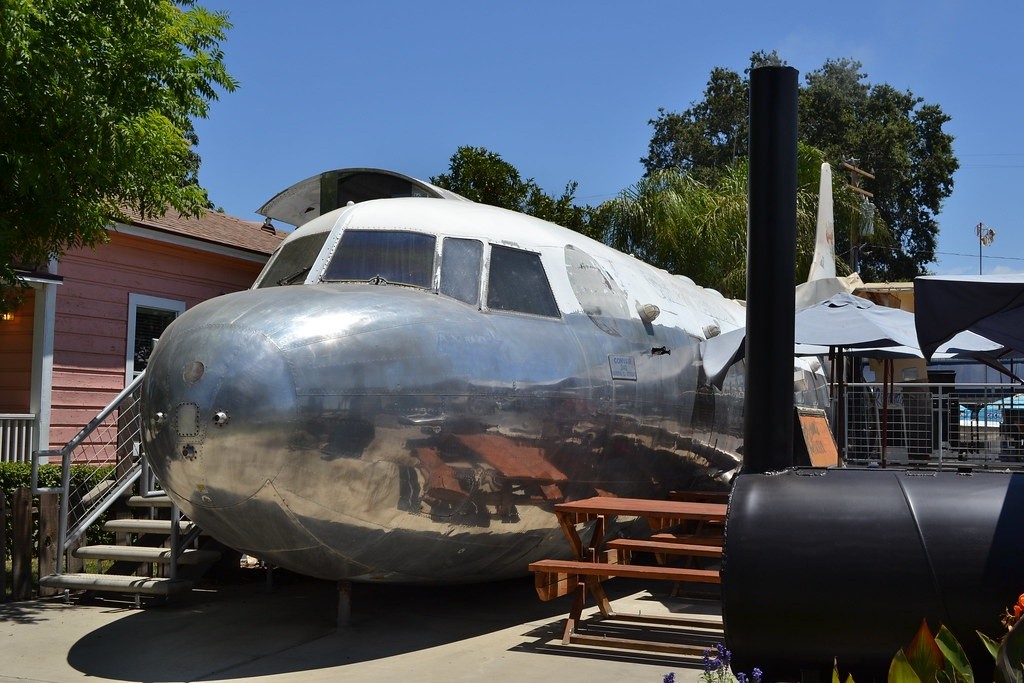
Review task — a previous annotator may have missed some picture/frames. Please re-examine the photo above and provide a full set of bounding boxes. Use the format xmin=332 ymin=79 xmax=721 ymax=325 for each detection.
xmin=794 ymin=406 xmax=847 ymax=469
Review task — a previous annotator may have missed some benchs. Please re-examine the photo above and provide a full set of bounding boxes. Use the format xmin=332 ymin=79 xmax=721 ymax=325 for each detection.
xmin=521 ymin=446 xmax=568 ymax=503
xmin=526 ymin=558 xmax=722 ymax=601
xmin=416 ymin=446 xmax=470 ymax=508
xmin=596 ymin=516 xmax=725 ymax=582
xmin=571 ymin=460 xmax=619 ymax=498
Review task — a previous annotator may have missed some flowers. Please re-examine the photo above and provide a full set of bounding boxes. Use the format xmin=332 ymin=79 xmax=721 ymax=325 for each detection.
xmin=660 ymin=642 xmax=763 ymax=682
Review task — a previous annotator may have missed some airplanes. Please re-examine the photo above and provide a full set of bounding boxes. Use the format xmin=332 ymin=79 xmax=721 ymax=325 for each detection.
xmin=140 ymin=159 xmax=840 ymax=595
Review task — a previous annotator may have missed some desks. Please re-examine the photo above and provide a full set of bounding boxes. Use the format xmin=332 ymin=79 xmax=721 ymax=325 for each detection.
xmin=659 ymin=490 xmax=730 ymax=600
xmin=553 ymin=495 xmax=728 ymax=656
xmin=451 ymin=432 xmax=568 ymax=523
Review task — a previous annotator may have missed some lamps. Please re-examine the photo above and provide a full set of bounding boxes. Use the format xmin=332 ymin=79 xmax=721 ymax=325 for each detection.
xmin=702 ymin=324 xmax=722 ymax=339
xmin=0 ymin=294 xmax=15 ymax=321
xmin=636 ymin=303 xmax=660 ymax=324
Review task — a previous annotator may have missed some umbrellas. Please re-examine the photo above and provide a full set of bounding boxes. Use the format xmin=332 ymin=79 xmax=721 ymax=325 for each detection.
xmin=697 ymin=270 xmax=1024 ymax=469
